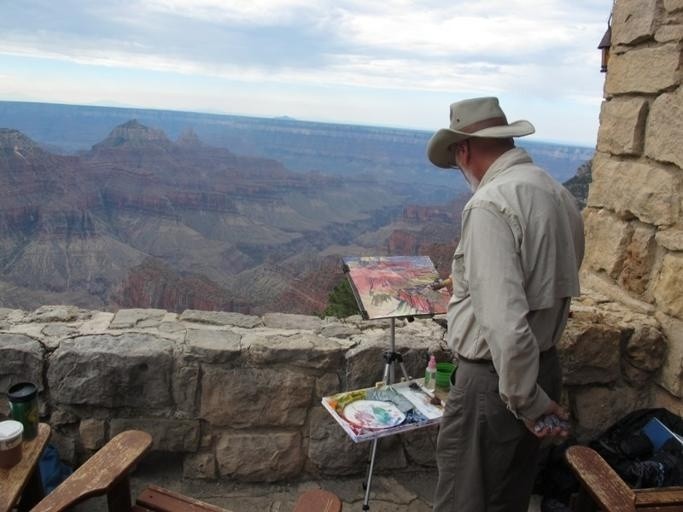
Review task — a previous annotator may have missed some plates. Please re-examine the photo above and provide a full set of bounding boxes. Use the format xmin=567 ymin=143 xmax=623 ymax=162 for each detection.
xmin=343 ymin=399 xmax=407 ymax=430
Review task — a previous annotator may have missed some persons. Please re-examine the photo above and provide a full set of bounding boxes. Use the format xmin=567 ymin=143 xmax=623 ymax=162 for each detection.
xmin=428 ymin=97 xmax=585 ymax=512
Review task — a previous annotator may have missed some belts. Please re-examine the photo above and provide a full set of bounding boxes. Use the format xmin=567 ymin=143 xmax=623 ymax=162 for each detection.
xmin=458 ymin=353 xmax=492 ymax=364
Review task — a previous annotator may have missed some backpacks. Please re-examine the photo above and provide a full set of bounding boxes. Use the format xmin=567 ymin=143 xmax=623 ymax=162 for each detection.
xmin=530 ymin=407 xmax=683 ymax=512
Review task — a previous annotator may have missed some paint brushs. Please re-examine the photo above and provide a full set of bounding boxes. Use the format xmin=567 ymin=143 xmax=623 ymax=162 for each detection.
xmin=434 ymin=279 xmax=451 ymax=291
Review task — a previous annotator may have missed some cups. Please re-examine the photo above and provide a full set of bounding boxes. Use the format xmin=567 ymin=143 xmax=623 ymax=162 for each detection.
xmin=0 ymin=420 xmax=25 ymax=469
xmin=7 ymin=380 xmax=39 ymax=440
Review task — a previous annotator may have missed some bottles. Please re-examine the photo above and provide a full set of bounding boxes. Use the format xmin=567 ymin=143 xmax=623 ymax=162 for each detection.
xmin=424 ymin=355 xmax=437 ymax=392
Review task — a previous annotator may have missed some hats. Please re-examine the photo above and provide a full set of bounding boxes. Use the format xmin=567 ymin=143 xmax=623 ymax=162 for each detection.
xmin=427 ymin=96 xmax=536 ymax=169
